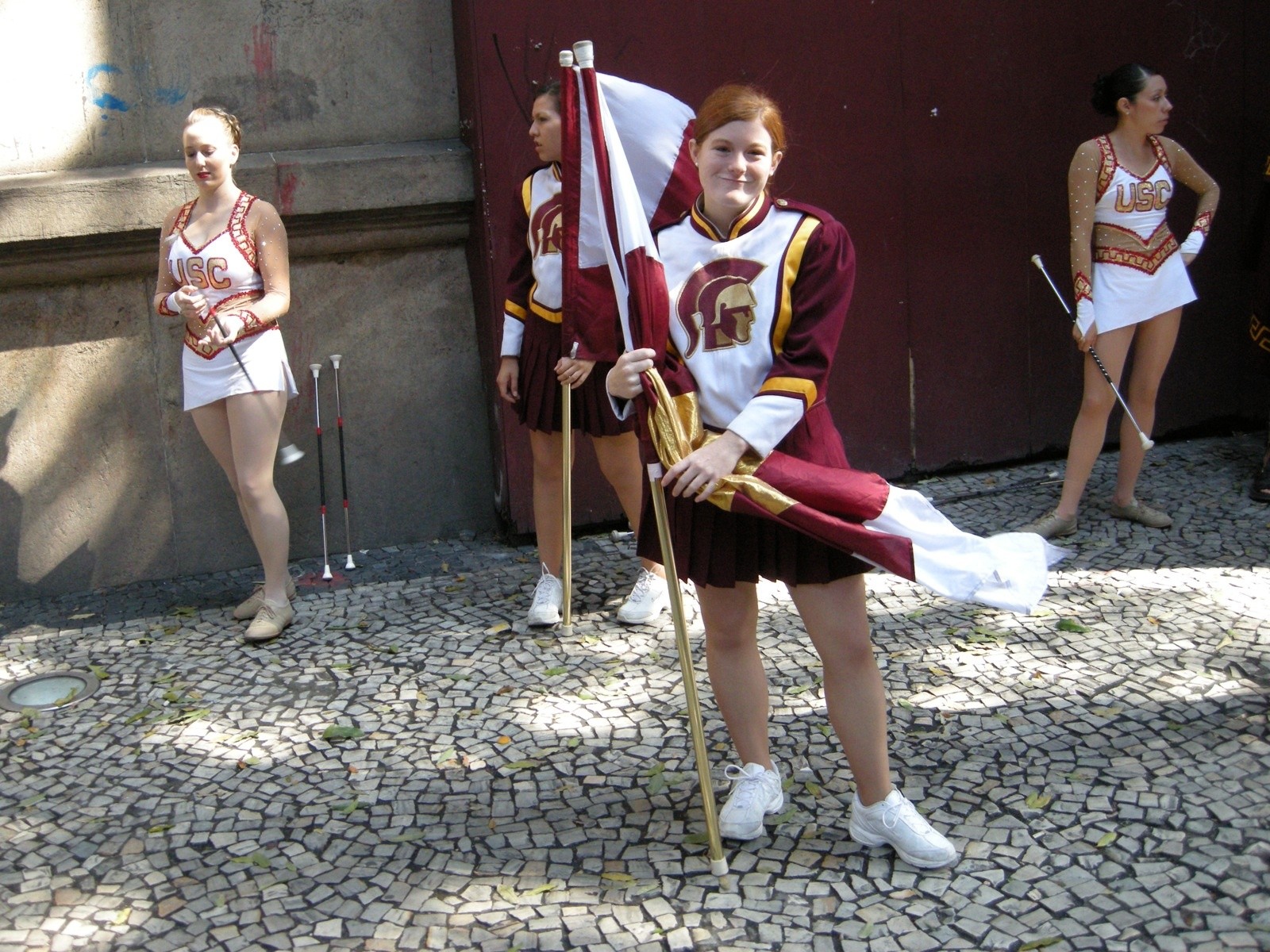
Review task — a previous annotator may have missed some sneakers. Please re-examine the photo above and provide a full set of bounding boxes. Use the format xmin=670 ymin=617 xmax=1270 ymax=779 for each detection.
xmin=528 ymin=561 xmax=563 ymax=624
xmin=719 ymin=759 xmax=783 ymax=840
xmin=849 ymin=782 xmax=957 ymax=868
xmin=244 ymin=596 xmax=295 ymax=640
xmin=233 ymin=576 xmax=297 ymax=620
xmin=616 ymin=563 xmax=672 ymax=624
xmin=1013 ymin=511 xmax=1077 ymax=541
xmin=1108 ymin=498 xmax=1173 ymax=527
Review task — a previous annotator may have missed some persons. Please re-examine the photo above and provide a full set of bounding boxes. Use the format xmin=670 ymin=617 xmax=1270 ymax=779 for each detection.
xmin=1012 ymin=59 xmax=1219 ymax=540
xmin=154 ymin=104 xmax=298 ymax=642
xmin=601 ymin=86 xmax=958 ymax=868
xmin=493 ymin=77 xmax=678 ymax=628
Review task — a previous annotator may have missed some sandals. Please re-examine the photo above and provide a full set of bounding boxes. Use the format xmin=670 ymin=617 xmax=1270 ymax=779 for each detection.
xmin=1249 ymin=468 xmax=1270 ymax=502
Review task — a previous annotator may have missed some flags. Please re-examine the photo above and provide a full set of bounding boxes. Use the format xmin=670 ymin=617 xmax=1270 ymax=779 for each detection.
xmin=552 ymin=67 xmax=625 ymax=368
xmin=579 ymin=62 xmax=1069 ymax=615
